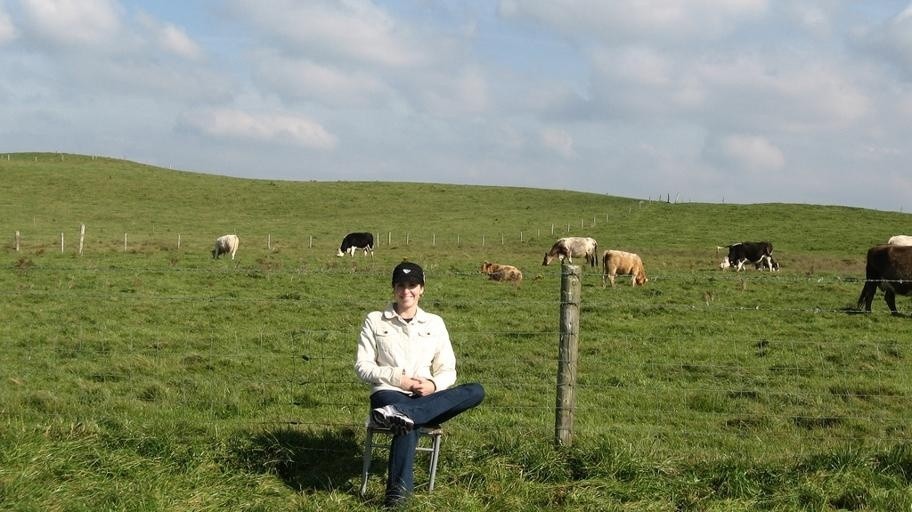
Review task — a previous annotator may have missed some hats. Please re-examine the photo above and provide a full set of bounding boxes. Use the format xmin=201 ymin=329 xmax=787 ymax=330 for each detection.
xmin=392 ymin=261 xmax=425 ymax=288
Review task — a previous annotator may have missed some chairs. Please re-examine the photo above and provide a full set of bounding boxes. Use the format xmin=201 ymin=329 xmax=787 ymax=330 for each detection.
xmin=360 ymin=383 xmax=443 ymax=497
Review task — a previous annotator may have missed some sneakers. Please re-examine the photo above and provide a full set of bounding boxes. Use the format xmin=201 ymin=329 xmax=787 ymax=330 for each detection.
xmin=372 ymin=404 xmax=414 ymax=437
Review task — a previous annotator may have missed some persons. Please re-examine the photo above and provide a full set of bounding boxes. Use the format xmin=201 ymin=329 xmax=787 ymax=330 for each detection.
xmin=355 ymin=261 xmax=486 ymax=508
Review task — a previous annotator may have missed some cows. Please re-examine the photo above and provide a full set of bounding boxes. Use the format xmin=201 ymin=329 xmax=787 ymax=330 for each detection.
xmin=210 ymin=234 xmax=240 ymax=260
xmin=857 ymin=234 xmax=912 ymax=312
xmin=719 ymin=240 xmax=780 ymax=273
xmin=542 ymin=237 xmax=599 ymax=269
xmin=602 ymin=249 xmax=648 ymax=289
xmin=335 ymin=232 xmax=374 ymax=260
xmin=480 ymin=261 xmax=524 ymax=283
